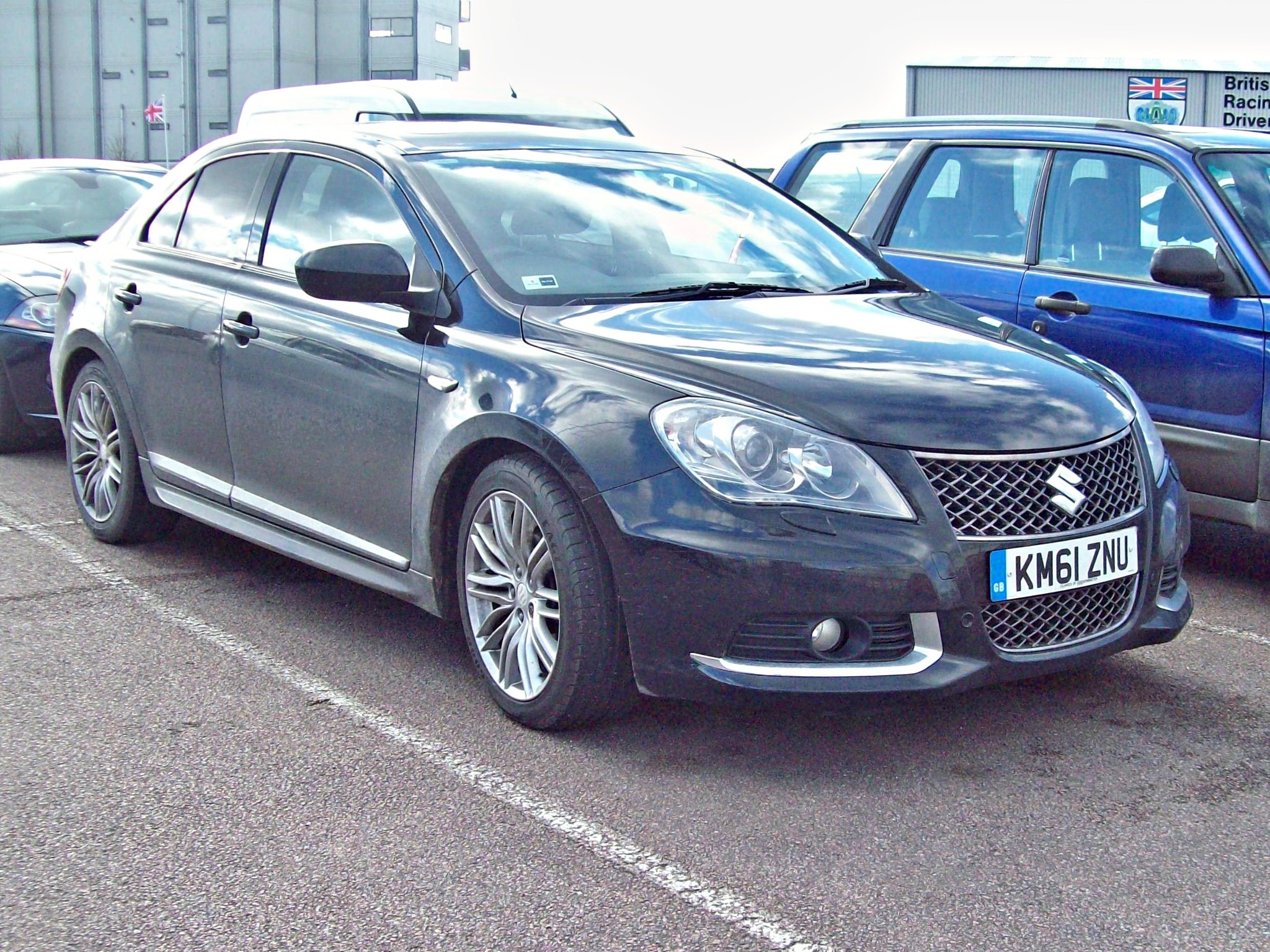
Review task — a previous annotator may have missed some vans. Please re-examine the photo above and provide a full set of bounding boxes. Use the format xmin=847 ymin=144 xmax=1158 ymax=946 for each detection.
xmin=229 ymin=80 xmax=706 ymax=263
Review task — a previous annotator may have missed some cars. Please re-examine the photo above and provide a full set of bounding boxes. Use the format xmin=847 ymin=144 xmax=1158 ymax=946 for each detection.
xmin=1135 ymin=178 xmax=1270 ymax=265
xmin=0 ymin=159 xmax=228 ymax=454
xmin=48 ymin=119 xmax=1196 ymax=734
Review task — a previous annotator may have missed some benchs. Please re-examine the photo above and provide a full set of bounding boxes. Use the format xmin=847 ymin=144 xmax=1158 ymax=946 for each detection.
xmin=921 ymin=193 xmax=1022 ymax=252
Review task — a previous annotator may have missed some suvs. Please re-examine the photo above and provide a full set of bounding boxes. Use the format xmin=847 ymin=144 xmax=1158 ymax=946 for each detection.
xmin=728 ymin=117 xmax=1270 ymax=534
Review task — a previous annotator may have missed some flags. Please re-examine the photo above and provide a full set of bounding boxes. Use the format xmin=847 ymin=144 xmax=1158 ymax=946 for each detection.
xmin=143 ymin=98 xmax=164 ymax=123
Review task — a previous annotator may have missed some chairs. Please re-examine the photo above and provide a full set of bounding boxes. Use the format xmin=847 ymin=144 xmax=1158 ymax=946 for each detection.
xmin=1067 ymin=175 xmax=1141 ymax=276
xmin=1157 ymin=179 xmax=1193 ymax=247
